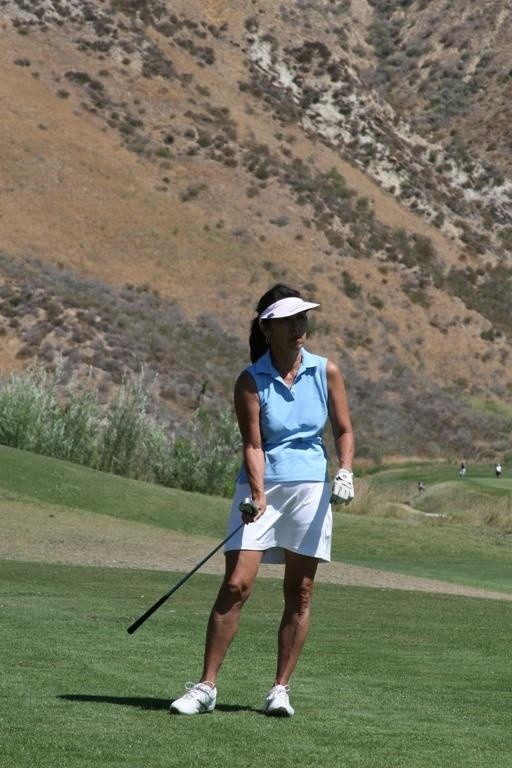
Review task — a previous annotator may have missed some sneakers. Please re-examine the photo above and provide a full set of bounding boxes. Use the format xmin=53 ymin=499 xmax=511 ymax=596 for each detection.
xmin=170 ymin=683 xmax=217 ymax=716
xmin=264 ymin=685 xmax=294 ymax=716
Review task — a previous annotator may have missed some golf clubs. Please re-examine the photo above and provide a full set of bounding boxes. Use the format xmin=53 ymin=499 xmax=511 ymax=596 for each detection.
xmin=126 ymin=498 xmax=258 ymax=634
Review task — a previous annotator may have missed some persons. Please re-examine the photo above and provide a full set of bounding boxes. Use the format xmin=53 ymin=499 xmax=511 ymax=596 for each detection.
xmin=495 ymin=462 xmax=502 ymax=478
xmin=458 ymin=460 xmax=467 ymax=478
xmin=167 ymin=281 xmax=355 ymax=717
xmin=418 ymin=479 xmax=425 ymax=494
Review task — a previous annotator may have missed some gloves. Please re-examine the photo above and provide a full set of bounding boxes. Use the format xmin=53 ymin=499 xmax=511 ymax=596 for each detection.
xmin=330 ymin=469 xmax=355 ymax=505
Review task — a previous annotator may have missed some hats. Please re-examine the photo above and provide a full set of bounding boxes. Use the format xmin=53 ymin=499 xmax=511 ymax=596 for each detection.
xmin=259 ymin=297 xmax=320 ymax=319
